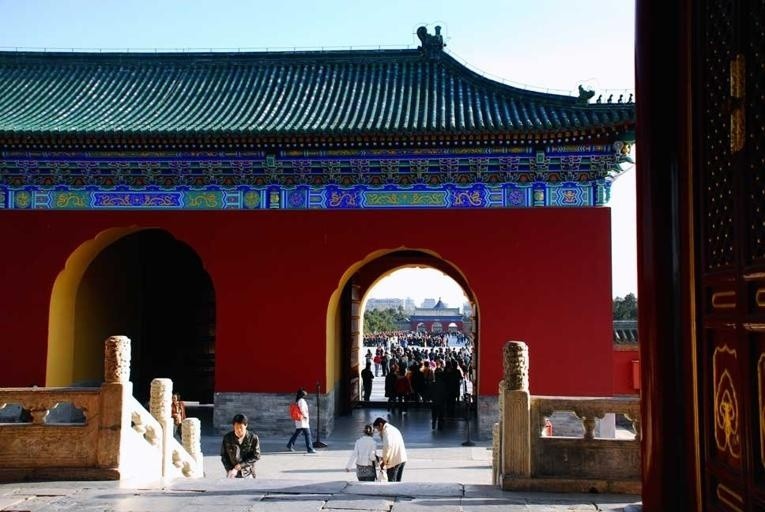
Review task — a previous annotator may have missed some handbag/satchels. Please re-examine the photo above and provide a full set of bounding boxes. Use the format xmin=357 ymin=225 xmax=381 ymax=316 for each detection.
xmin=173 ymin=414 xmax=183 ymax=424
xmin=374 ymin=463 xmax=388 ymax=483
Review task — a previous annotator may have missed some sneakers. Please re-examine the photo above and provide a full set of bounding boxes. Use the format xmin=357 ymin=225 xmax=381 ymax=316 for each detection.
xmin=308 ymin=449 xmax=319 ymax=453
xmin=286 ymin=445 xmax=297 ymax=452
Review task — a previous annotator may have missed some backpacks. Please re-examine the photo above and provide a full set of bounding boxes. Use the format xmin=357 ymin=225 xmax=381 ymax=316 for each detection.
xmin=289 ymin=398 xmax=304 ymax=422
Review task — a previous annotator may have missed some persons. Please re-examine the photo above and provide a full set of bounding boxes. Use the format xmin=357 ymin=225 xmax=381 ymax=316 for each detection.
xmin=220 ymin=413 xmax=260 ymax=479
xmin=286 ymin=387 xmax=318 ymax=453
xmin=361 ymin=329 xmax=473 ymax=430
xmin=373 ymin=417 xmax=407 ymax=482
xmin=344 ymin=424 xmax=381 ymax=481
